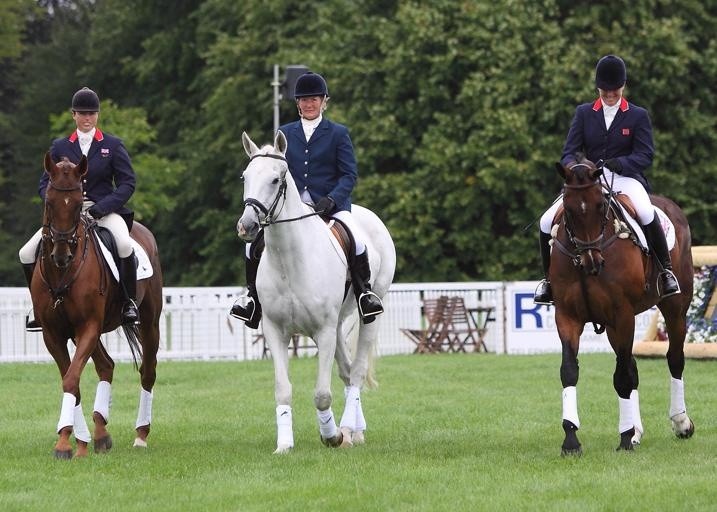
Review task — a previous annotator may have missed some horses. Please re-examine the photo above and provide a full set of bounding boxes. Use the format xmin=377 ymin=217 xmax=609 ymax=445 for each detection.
xmin=29 ymin=149 xmax=163 ymax=463
xmin=547 ymin=159 xmax=696 ymax=462
xmin=236 ymin=129 xmax=397 ymax=456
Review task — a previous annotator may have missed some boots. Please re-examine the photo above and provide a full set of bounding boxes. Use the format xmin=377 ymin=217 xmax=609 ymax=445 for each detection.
xmin=346 ymin=244 xmax=382 ymax=324
xmin=641 ymin=209 xmax=678 ymax=295
xmin=534 ymin=228 xmax=553 ymax=302
xmin=118 ymin=248 xmax=137 ymax=319
xmin=232 ymin=255 xmax=262 ymax=329
xmin=21 ymin=262 xmax=43 ymax=331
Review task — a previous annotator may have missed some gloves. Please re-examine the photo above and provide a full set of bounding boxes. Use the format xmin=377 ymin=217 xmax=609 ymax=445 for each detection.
xmin=604 ymin=158 xmax=623 ymax=175
xmin=89 ymin=209 xmax=104 ymax=219
xmin=317 ymin=197 xmax=335 ymax=215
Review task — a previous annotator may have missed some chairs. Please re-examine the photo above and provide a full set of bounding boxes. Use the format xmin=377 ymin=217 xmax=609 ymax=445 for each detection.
xmin=402 ymin=295 xmax=490 ymax=352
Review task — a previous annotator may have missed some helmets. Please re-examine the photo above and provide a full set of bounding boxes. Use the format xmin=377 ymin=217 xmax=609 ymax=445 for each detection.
xmin=71 ymin=86 xmax=101 ymax=113
xmin=596 ymin=55 xmax=627 ymax=91
xmin=294 ymin=72 xmax=328 ymax=97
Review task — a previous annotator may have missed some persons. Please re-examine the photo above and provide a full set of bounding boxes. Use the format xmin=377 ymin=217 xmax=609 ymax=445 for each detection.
xmin=530 ymin=54 xmax=686 ymax=308
xmin=17 ymin=86 xmax=156 ymax=332
xmin=226 ymin=69 xmax=387 ymax=329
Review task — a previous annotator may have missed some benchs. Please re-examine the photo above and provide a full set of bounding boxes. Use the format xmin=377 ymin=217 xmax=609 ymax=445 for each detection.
xmin=251 ymin=328 xmax=316 ymax=364
